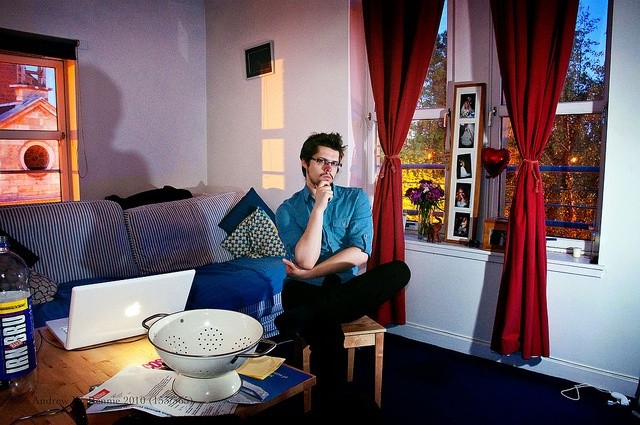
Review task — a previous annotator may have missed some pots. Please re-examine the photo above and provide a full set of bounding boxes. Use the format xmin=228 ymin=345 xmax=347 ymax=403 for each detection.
xmin=142 ymin=308 xmax=278 ymax=402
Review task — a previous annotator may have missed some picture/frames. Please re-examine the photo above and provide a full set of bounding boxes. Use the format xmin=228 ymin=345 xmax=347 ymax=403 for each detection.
xmin=243 ymin=40 xmax=275 ymax=81
xmin=483 ymin=220 xmax=507 ymax=254
xmin=444 ymin=82 xmax=487 ymax=247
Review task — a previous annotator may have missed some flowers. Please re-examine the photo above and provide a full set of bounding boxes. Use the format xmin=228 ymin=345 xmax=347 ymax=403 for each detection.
xmin=404 ymin=179 xmax=445 ymax=208
xmin=588 ymin=225 xmax=597 ymax=240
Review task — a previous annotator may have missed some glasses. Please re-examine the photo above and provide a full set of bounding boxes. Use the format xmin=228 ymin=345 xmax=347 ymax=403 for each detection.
xmin=311 ymin=157 xmax=343 ymax=168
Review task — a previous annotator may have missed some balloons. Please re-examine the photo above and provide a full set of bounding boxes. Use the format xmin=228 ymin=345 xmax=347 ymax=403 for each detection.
xmin=482 ymin=148 xmax=511 ymax=178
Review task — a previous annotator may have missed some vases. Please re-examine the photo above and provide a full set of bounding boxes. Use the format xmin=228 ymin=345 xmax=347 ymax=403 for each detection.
xmin=417 ymin=204 xmax=433 ymax=241
xmin=591 ymin=239 xmax=596 ymax=260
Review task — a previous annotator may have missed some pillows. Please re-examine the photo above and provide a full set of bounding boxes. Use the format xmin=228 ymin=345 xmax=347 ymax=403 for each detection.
xmin=219 ymin=205 xmax=289 ymax=258
xmin=217 ymin=186 xmax=276 ymax=235
xmin=0 ymin=226 xmax=39 ymax=268
xmin=0 ymin=254 xmax=57 ymax=305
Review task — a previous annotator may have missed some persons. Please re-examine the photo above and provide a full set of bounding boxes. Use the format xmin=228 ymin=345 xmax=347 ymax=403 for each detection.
xmin=458 ymin=217 xmax=468 ymax=234
xmin=274 ymin=133 xmax=410 ymax=425
xmin=461 ymin=97 xmax=473 ymax=118
xmin=457 ymin=186 xmax=469 ymax=207
xmin=461 ymin=124 xmax=472 ymax=146
xmin=459 ymin=156 xmax=471 ymax=178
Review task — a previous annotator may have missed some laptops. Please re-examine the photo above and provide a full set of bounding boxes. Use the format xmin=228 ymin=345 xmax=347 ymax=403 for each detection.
xmin=45 ymin=268 xmax=198 ymax=353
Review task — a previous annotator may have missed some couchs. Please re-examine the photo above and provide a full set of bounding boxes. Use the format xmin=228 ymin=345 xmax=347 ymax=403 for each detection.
xmin=0 ymin=186 xmax=287 ymax=342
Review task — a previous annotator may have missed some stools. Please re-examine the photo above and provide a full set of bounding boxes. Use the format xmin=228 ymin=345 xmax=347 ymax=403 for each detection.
xmin=301 ymin=311 xmax=388 ymax=414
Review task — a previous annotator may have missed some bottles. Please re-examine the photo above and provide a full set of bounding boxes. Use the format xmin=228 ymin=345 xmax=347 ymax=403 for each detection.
xmin=0 ymin=235 xmax=39 ymax=399
